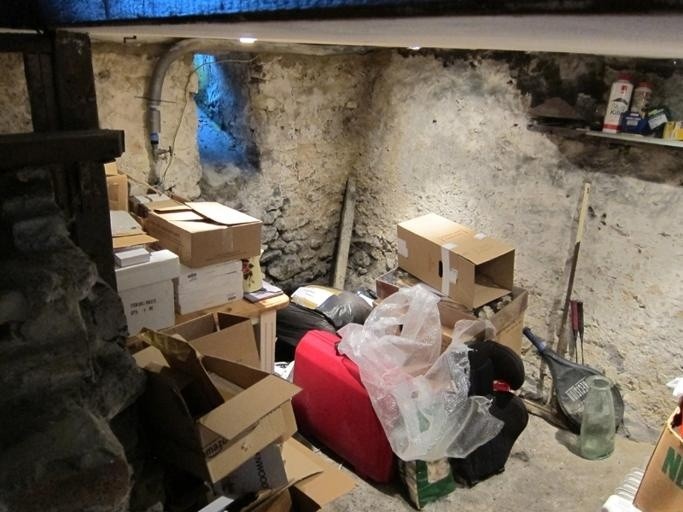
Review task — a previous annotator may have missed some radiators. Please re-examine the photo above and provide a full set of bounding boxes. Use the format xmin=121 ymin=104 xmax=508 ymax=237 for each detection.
xmin=601 ymin=467 xmax=645 ymax=512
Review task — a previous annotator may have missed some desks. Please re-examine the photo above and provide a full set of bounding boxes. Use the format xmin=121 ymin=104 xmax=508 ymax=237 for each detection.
xmin=174 ymin=278 xmax=290 ymax=375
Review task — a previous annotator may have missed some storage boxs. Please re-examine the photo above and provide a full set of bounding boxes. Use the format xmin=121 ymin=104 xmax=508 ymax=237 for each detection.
xmin=143 ymin=352 xmax=357 ymax=512
xmin=104 ymin=163 xmax=262 ymax=334
xmin=154 ymin=313 xmax=262 ymax=370
xmin=632 ymin=401 xmax=683 ymax=512
xmin=371 ymin=211 xmax=529 ymax=358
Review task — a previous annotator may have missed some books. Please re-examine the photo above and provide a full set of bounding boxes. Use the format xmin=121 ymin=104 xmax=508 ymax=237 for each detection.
xmin=113 ymin=247 xmax=150 ymax=267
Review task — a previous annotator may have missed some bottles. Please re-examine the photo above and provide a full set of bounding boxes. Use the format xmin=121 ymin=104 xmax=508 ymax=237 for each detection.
xmin=602 ymin=73 xmax=634 ymax=134
xmin=629 ymin=80 xmax=654 ymax=116
xmin=578 ymin=374 xmax=617 ymax=460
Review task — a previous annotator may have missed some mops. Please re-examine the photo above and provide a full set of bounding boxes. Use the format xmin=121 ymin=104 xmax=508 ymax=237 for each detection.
xmin=518 ymin=181 xmax=591 ymax=430
